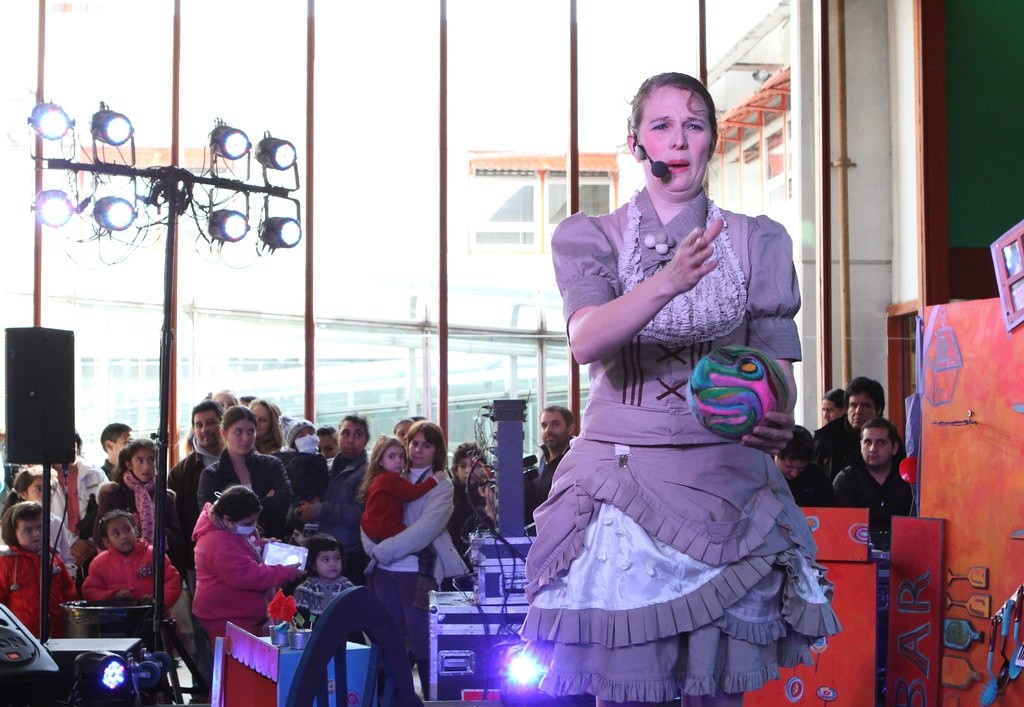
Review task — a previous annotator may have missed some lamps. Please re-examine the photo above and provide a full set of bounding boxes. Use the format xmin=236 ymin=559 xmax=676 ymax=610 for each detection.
xmin=208 ymin=125 xmax=302 ymax=249
xmin=26 ymin=102 xmax=138 ymax=232
xmin=65 ymin=651 xmax=128 ymax=707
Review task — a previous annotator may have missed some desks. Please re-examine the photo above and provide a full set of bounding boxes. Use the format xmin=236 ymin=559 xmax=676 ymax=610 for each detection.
xmin=211 ymin=621 xmax=378 ymax=707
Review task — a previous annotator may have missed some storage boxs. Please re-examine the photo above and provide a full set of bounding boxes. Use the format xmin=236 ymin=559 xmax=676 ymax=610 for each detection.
xmin=469 ymin=534 xmax=539 ymax=606
xmin=427 ymin=589 xmax=596 ymax=707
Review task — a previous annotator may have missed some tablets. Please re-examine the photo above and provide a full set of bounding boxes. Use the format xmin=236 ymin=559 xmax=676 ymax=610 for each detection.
xmin=262 ymin=542 xmax=308 ymax=571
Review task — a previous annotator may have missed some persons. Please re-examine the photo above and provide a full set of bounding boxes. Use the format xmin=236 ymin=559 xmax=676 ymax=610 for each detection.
xmin=517 ymin=72 xmax=841 ymax=707
xmin=0 ymin=384 xmax=493 ymax=702
xmin=523 ymin=406 xmax=580 ymax=541
xmin=773 ymin=376 xmax=918 ymax=553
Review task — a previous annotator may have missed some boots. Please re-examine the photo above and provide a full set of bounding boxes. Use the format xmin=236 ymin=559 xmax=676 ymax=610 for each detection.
xmin=414 ymin=575 xmax=439 ymax=611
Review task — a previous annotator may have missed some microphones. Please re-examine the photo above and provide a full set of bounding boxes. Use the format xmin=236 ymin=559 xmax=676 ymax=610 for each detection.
xmin=635 ymin=144 xmax=668 ymax=177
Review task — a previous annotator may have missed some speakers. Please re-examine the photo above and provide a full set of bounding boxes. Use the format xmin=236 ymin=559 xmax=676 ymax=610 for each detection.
xmin=4 ymin=326 xmax=75 ymax=466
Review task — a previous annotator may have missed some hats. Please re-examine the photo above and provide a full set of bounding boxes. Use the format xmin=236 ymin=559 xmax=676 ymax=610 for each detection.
xmin=280 ymin=415 xmax=315 ymax=449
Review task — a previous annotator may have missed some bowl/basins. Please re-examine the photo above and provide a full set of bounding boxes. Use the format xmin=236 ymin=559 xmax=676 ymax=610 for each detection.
xmin=60 ymin=599 xmax=152 ymax=626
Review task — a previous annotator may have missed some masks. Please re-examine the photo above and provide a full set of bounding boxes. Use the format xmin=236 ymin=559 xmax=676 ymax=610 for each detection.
xmin=231 ymin=522 xmax=257 ymax=535
xmin=293 ymin=434 xmax=319 ymax=454
